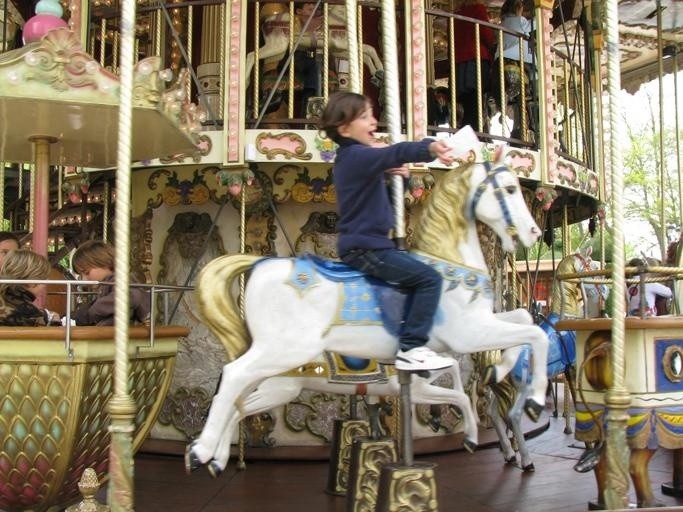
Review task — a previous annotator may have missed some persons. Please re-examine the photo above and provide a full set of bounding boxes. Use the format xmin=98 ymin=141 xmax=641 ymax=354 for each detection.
xmin=323 ymin=92 xmax=458 ymax=374
xmin=0 ymin=231 xmax=23 ymax=275
xmin=0 ymin=249 xmax=60 ymax=326
xmin=488 ymin=1 xmax=541 ymax=154
xmin=445 ymin=1 xmax=499 ymax=138
xmin=69 ymin=241 xmax=155 ymax=328
xmin=625 ymin=261 xmax=673 ymax=318
xmin=276 ymin=1 xmax=319 ymax=118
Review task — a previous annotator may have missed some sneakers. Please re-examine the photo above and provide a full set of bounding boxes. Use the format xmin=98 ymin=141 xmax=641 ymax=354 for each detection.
xmin=393 ymin=345 xmax=457 ymax=371
